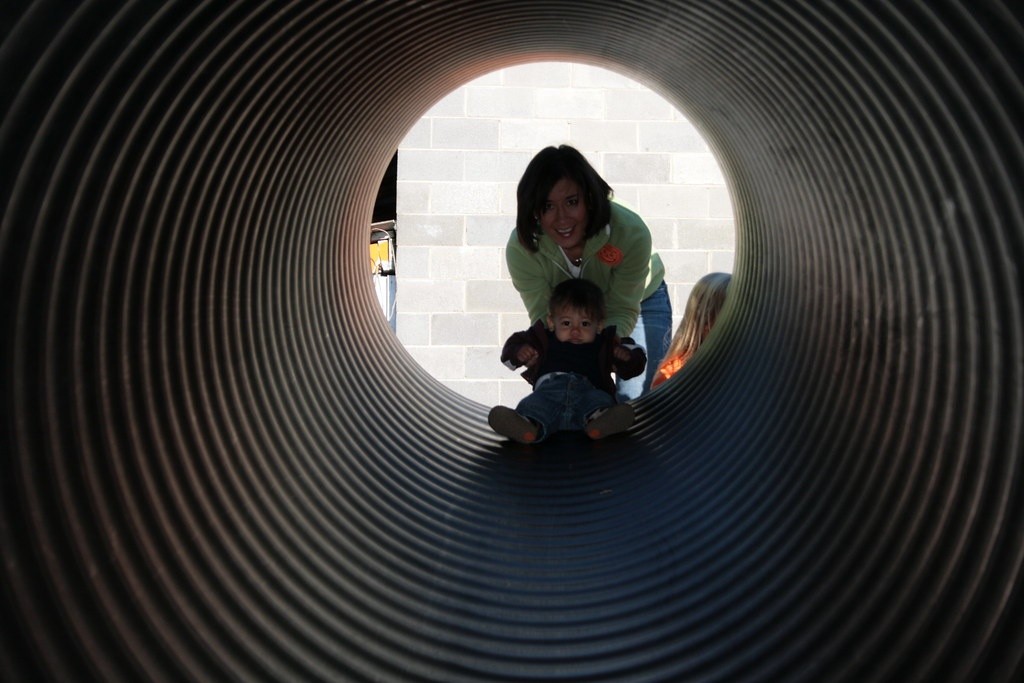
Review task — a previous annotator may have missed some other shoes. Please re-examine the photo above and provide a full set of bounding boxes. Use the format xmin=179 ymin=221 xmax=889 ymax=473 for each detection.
xmin=488 ymin=406 xmax=539 ymax=445
xmin=585 ymin=404 xmax=635 ymax=439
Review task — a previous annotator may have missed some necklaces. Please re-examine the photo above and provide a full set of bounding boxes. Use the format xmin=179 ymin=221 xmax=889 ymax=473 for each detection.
xmin=566 ymin=254 xmax=583 ymax=263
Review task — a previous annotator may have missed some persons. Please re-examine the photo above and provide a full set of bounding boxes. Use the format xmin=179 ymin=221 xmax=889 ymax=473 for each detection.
xmin=506 ymin=146 xmax=671 ymax=405
xmin=488 ymin=279 xmax=646 ymax=445
xmin=650 ymin=273 xmax=736 ymax=392
